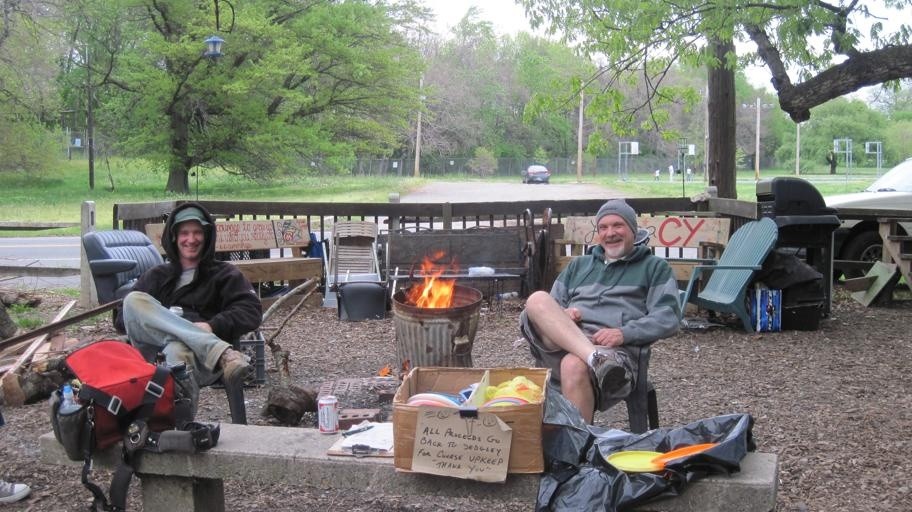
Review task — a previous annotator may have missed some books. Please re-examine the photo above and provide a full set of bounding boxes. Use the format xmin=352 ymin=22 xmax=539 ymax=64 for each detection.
xmin=328 ymin=436 xmax=394 ymax=458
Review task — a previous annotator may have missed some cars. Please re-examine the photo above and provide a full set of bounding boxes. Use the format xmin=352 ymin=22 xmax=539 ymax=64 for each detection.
xmin=823 ymin=157 xmax=912 ymax=287
xmin=522 ymin=165 xmax=551 ymax=184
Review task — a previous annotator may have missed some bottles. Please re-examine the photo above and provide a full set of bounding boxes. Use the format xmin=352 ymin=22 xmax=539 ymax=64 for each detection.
xmin=57 ymin=385 xmax=88 ymax=464
xmin=495 ymin=291 xmax=519 ymax=302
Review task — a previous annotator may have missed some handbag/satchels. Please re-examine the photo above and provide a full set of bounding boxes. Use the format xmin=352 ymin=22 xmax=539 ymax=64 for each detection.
xmin=51 ymin=340 xmax=192 ymax=461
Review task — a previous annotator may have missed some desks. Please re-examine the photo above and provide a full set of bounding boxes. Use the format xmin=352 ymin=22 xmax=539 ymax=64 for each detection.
xmin=822 ymin=190 xmax=910 ymax=307
xmin=388 ymin=273 xmax=521 ymax=323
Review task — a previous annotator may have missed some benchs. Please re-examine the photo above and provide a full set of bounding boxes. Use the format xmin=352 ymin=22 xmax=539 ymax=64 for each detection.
xmin=551 ymin=214 xmax=729 ymax=323
xmin=38 ymin=420 xmax=777 ymax=512
xmin=142 ymin=219 xmax=323 ymax=310
xmin=378 ymin=228 xmax=537 ymax=299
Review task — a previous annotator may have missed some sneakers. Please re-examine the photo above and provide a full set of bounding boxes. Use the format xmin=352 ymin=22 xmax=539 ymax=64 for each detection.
xmin=0 ymin=479 xmax=31 ymax=503
xmin=592 ymin=354 xmax=630 ymax=395
xmin=220 ymin=349 xmax=256 ymax=384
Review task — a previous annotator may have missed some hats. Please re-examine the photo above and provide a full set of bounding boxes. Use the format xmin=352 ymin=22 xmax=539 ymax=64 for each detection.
xmin=595 ymin=199 xmax=637 ymax=239
xmin=170 ymin=207 xmax=211 ymax=243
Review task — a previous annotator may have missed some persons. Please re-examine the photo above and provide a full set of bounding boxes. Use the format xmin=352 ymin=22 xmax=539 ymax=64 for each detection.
xmin=517 ymin=200 xmax=684 ymax=431
xmin=110 ymin=200 xmax=265 ymax=425
xmin=1 ymin=411 xmax=32 ymax=508
xmin=668 ymin=164 xmax=674 ymax=180
xmin=655 ymin=169 xmax=660 ymax=181
xmin=686 ymin=166 xmax=692 ymax=181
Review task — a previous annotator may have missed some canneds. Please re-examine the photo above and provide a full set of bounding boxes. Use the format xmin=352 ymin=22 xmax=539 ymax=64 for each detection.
xmin=170 ymin=306 xmax=184 ymax=318
xmin=317 ymin=396 xmax=339 ymax=434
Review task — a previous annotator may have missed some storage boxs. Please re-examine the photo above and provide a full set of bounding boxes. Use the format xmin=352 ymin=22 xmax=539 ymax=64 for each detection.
xmin=390 ymin=365 xmax=549 ymax=477
xmin=746 ymin=286 xmax=783 ymax=334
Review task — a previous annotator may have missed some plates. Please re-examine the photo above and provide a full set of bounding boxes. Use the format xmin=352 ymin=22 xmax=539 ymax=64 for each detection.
xmin=605 ymin=443 xmax=720 ymax=473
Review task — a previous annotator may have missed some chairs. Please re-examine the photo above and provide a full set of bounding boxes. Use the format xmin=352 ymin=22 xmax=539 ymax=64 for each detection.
xmin=319 ymin=219 xmax=401 ymax=322
xmin=83 ymin=229 xmax=165 ymax=309
xmin=676 ymin=216 xmax=778 ymax=336
